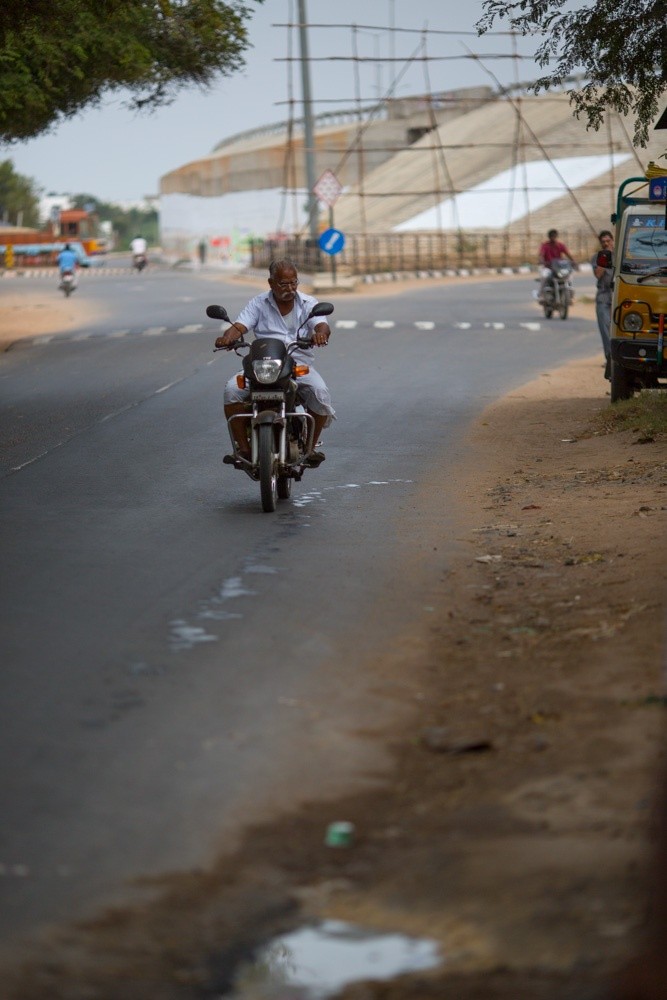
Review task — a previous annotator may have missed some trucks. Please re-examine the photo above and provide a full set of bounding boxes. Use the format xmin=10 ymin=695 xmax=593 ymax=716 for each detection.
xmin=596 ymin=176 xmax=667 ymax=403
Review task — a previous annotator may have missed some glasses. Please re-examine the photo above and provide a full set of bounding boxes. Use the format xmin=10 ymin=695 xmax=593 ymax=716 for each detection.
xmin=275 ymin=280 xmax=300 ymax=289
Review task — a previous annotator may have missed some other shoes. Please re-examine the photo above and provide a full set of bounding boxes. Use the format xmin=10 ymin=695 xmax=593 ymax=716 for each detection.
xmin=223 ymin=451 xmax=253 ymax=466
xmin=305 ymin=450 xmax=326 ymax=467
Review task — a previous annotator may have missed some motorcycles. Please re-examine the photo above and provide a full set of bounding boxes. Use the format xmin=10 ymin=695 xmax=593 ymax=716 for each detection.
xmin=57 ymin=259 xmax=80 ymax=298
xmin=130 ymin=248 xmax=146 ymax=271
xmin=206 ymin=302 xmax=335 ymax=512
xmin=534 ymin=257 xmax=577 ymax=320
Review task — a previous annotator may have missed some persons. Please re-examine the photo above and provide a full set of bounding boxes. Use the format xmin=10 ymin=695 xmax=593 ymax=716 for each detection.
xmin=130 ymin=235 xmax=147 ymax=268
xmin=215 ymin=258 xmax=337 ymax=467
xmin=536 ymin=230 xmax=579 ymax=305
xmin=198 ymin=241 xmax=206 ymax=265
xmin=54 ymin=244 xmax=80 ymax=290
xmin=591 ymin=231 xmax=616 ymax=380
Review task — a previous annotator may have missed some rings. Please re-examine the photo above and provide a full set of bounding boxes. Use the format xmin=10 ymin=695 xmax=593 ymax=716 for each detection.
xmin=320 ymin=335 xmax=324 ymax=338
xmin=218 ymin=341 xmax=222 ymax=344
